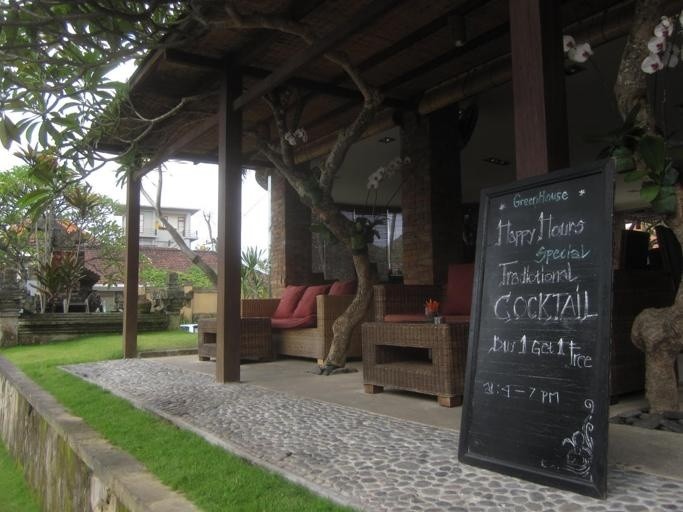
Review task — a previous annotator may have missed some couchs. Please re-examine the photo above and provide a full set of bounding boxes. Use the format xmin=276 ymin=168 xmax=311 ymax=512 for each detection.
xmin=241 ymin=295 xmax=375 ymax=365
xmin=375 ymin=284 xmax=675 ymax=403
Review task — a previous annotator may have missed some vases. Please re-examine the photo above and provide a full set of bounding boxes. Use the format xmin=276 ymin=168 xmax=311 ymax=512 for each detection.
xmin=612 ymin=148 xmax=636 ymax=172
xmin=350 ymin=236 xmax=365 ymax=252
xmin=651 ymin=187 xmax=676 ymax=215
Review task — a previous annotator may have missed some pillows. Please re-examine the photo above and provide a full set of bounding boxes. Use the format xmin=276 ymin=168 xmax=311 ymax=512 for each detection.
xmin=273 ymin=285 xmax=307 ymax=318
xmin=441 ymin=263 xmax=473 ymax=316
xmin=328 ymin=279 xmax=356 ymax=295
xmin=292 ymin=284 xmax=330 ymax=318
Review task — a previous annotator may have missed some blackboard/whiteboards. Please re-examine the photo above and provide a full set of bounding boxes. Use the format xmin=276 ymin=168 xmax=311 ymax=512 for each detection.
xmin=457 ymin=157 xmax=614 ymax=500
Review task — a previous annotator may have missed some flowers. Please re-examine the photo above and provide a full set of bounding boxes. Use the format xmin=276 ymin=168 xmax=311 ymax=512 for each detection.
xmin=559 ymin=34 xmax=649 ymax=158
xmin=624 ymin=11 xmax=683 ymax=200
xmin=355 ymin=156 xmax=412 ymax=244
